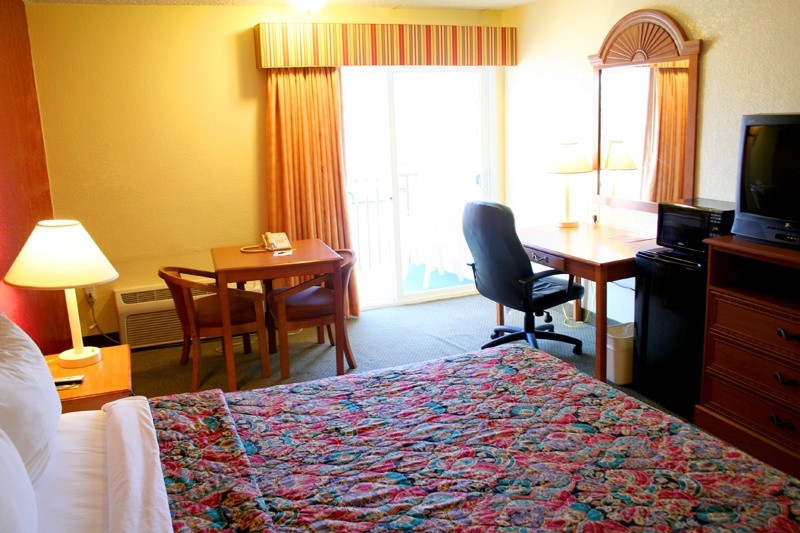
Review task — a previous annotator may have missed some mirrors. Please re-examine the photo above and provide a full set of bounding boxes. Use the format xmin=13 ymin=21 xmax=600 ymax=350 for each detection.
xmin=588 ymin=8 xmax=703 ymax=209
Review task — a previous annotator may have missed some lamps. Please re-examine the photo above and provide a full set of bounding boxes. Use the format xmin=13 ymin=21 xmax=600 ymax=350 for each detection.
xmin=601 ymin=135 xmax=637 ymax=203
xmin=545 ymin=142 xmax=592 ymax=229
xmin=4 ymin=218 xmax=120 ymax=368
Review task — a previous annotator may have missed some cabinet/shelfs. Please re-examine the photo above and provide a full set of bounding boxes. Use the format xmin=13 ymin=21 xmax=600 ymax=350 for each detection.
xmin=692 ymin=237 xmax=800 ymax=481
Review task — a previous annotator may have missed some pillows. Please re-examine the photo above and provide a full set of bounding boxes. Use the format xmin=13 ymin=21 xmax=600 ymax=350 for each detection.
xmin=1 ymin=427 xmax=40 ymax=533
xmin=1 ymin=311 xmax=60 ymax=485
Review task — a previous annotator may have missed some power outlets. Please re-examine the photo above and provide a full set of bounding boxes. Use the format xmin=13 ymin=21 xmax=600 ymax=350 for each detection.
xmin=81 ymin=287 xmax=96 ymax=307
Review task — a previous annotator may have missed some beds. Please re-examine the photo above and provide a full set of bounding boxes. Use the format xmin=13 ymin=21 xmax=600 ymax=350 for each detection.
xmin=39 ymin=345 xmax=799 ymax=532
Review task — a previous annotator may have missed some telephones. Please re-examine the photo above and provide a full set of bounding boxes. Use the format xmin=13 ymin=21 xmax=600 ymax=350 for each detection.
xmin=261 ymin=232 xmax=292 ymax=251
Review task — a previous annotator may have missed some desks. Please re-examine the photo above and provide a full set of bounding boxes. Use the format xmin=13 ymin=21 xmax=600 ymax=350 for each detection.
xmin=43 ymin=343 xmax=133 ymax=415
xmin=511 ymin=206 xmax=663 ymax=382
xmin=211 ymin=237 xmax=344 ymax=391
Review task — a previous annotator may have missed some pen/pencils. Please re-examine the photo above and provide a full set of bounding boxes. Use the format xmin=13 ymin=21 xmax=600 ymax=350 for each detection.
xmin=278 ymin=249 xmax=296 ymax=254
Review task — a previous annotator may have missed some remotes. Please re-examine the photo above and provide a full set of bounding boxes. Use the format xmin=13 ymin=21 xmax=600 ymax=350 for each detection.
xmin=55 ymin=375 xmax=84 ymax=385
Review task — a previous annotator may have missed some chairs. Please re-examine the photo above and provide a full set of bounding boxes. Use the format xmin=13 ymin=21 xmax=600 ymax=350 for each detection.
xmin=461 ymin=199 xmax=585 ymax=357
xmin=265 ymin=248 xmax=358 ymax=379
xmin=157 ymin=267 xmax=270 ymax=392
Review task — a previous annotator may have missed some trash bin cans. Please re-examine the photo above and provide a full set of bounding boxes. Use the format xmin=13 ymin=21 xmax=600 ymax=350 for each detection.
xmin=606 ymin=322 xmax=634 ymax=385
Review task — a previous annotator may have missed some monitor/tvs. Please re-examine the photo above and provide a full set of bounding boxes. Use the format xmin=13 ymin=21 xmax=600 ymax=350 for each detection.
xmin=730 ymin=114 xmax=800 ymax=247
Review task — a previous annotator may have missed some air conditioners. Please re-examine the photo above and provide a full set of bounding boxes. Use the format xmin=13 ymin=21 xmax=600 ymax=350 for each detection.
xmin=114 ymin=279 xmax=264 ymax=349
